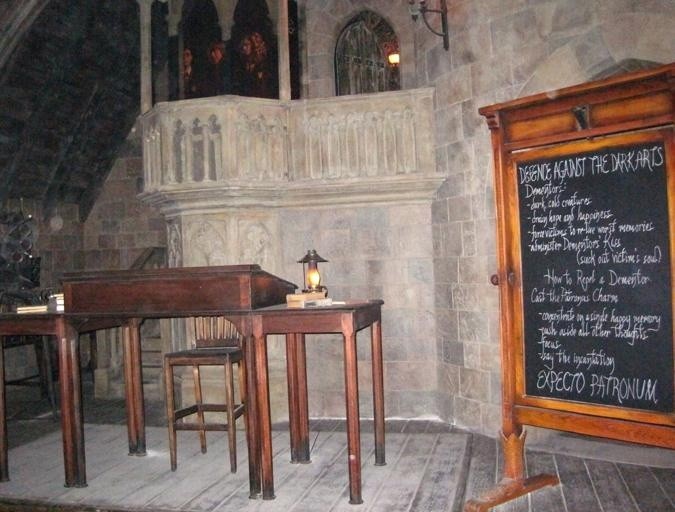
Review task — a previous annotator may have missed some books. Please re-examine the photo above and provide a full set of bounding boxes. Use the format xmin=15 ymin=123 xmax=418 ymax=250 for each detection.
xmin=15 ymin=294 xmax=64 ymax=314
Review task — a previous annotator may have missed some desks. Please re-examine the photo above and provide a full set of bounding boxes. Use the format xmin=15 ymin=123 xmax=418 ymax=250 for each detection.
xmin=0 ymin=264 xmax=385 ymax=505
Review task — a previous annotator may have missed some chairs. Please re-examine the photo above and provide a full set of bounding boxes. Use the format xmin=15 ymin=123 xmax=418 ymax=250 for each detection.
xmin=163 ymin=311 xmax=248 ymax=472
xmin=0 ymin=290 xmax=59 ymax=423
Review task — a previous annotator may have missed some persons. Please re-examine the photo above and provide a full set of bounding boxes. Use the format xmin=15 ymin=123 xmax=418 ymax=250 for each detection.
xmin=169 ymin=32 xmax=268 ymax=102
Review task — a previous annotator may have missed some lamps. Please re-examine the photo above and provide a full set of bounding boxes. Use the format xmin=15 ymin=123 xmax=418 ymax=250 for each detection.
xmin=297 ymin=248 xmax=328 ymax=293
xmin=408 ymin=1 xmax=450 ymax=51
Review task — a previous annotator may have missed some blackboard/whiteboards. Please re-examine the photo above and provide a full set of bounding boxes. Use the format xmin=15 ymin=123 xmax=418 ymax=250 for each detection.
xmin=509 ymin=125 xmax=675 ymax=429
